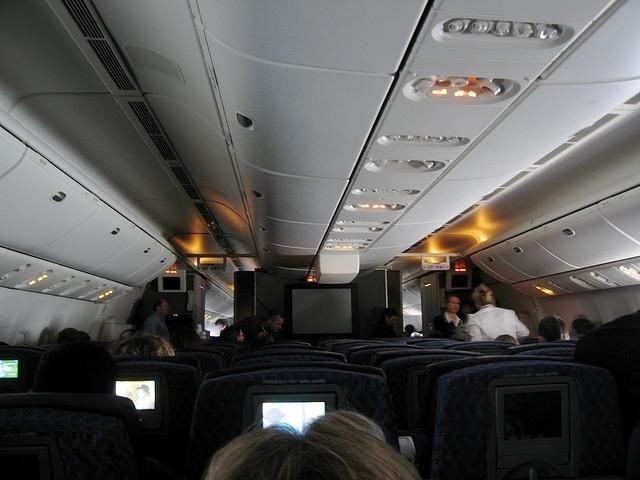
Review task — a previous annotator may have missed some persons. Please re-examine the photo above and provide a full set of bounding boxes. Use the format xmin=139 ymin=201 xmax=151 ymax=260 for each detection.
xmin=33 ymin=298 xmax=287 ymax=394
xmin=202 ymin=409 xmax=422 ymax=480
xmin=371 ymin=284 xmax=597 ymax=344
xmin=134 ymin=386 xmax=149 ymax=410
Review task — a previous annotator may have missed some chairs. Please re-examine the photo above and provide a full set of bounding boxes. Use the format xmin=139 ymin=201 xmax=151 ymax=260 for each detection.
xmin=1 ymin=327 xmax=638 ymax=476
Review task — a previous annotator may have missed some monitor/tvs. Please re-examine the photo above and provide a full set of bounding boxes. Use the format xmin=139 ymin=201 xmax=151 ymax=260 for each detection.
xmin=108 ymin=372 xmax=168 ymax=430
xmin=0 ymin=353 xmax=24 ymax=384
xmin=446 ymin=271 xmax=472 ymax=291
xmin=287 ymin=283 xmax=356 ymax=339
xmin=158 ymin=270 xmax=186 ymax=292
xmin=413 ymin=370 xmax=425 ymax=425
xmin=495 ymin=384 xmax=569 ymax=469
xmin=0 ymin=446 xmax=51 ymax=480
xmin=252 ymin=393 xmax=338 ymax=436
